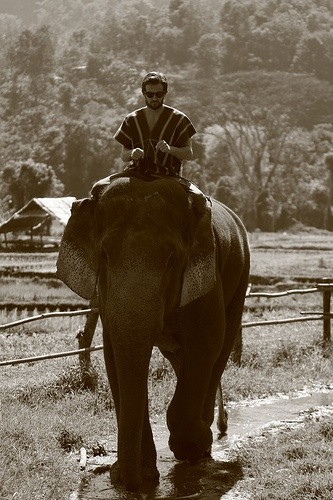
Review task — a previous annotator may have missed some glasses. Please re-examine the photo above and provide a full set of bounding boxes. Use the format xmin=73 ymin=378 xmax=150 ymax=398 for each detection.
xmin=145 ymin=91 xmax=165 ymax=98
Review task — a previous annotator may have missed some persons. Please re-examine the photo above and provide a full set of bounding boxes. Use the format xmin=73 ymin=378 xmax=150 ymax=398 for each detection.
xmin=92 ymin=71 xmax=198 ymax=190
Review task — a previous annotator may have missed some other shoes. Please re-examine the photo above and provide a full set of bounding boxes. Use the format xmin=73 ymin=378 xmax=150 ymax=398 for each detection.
xmin=80 ymin=198 xmax=94 ymax=213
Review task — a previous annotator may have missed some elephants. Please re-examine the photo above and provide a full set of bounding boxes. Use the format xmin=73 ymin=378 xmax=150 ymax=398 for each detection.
xmin=55 ymin=176 xmax=251 ymax=489
